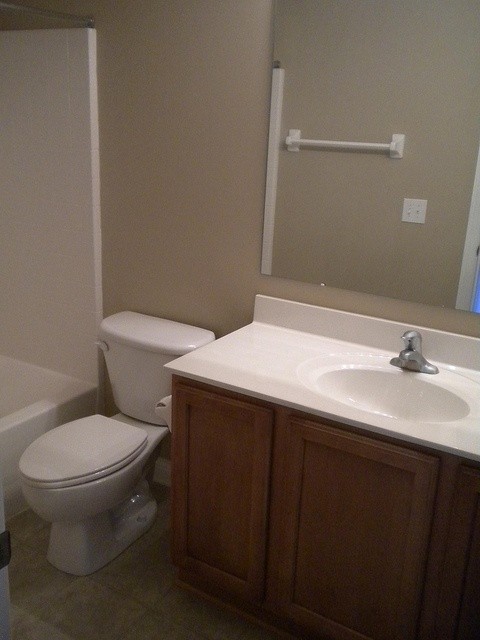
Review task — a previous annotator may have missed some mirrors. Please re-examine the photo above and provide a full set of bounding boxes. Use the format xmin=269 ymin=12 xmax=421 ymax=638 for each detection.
xmin=261 ymin=2 xmax=480 ymax=313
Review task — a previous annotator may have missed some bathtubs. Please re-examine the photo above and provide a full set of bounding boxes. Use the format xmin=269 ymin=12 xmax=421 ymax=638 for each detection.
xmin=0 ymin=353 xmax=99 ymax=521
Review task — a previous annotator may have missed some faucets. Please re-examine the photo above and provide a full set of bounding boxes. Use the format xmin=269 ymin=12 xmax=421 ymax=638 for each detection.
xmin=391 ymin=331 xmax=438 ymax=373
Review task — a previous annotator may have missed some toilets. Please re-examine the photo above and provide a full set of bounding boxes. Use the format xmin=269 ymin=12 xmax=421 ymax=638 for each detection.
xmin=19 ymin=310 xmax=215 ymax=576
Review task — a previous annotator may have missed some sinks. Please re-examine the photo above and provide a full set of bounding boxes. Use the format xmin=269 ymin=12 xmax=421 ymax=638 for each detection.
xmin=313 ymin=364 xmax=480 ymax=422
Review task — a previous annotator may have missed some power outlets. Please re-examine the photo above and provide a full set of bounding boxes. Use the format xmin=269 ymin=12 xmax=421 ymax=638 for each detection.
xmin=402 ymin=199 xmax=427 ymax=224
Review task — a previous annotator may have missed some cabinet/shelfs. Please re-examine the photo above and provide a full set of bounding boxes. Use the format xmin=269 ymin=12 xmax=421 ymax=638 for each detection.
xmin=162 ymin=367 xmax=479 ymax=640
